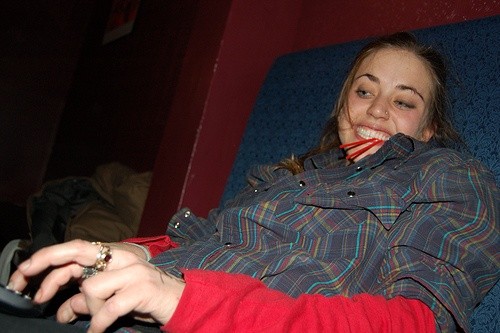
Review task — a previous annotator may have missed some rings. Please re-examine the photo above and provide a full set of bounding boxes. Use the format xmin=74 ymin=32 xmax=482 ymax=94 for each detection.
xmin=80 ymin=241 xmax=113 ymax=279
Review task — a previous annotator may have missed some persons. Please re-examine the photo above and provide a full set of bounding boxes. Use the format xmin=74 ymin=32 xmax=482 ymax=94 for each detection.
xmin=0 ymin=33 xmax=500 ymax=333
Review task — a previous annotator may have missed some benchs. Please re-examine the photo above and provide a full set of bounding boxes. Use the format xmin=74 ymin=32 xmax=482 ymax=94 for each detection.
xmin=220 ymin=15 xmax=499 ymax=332
